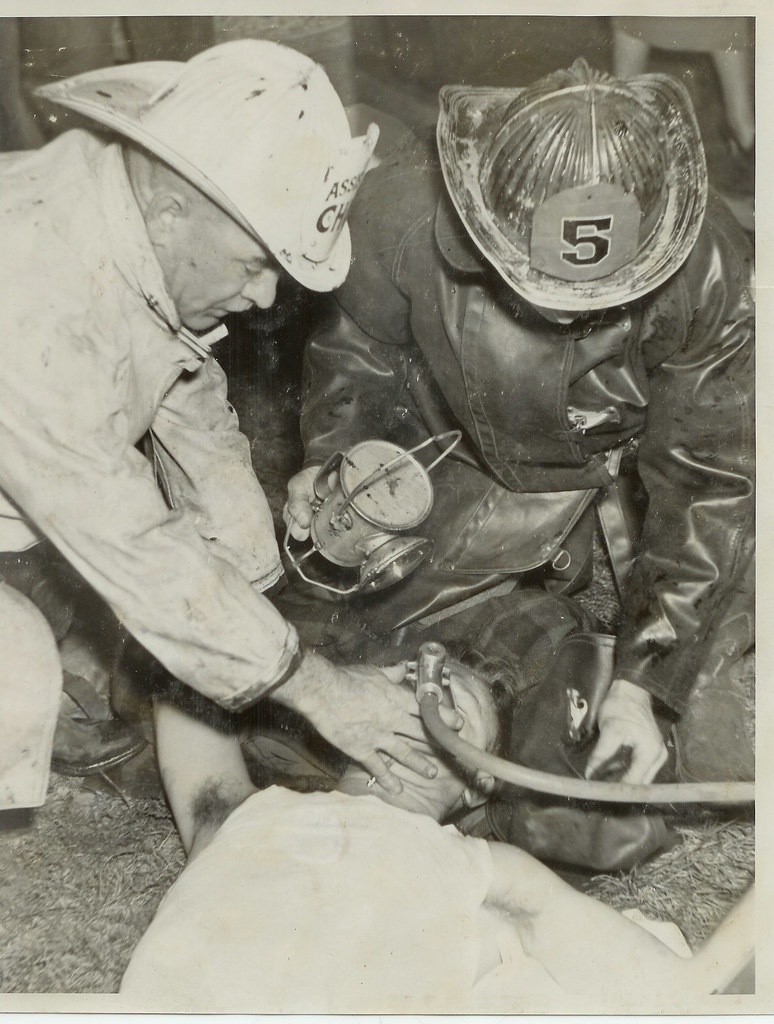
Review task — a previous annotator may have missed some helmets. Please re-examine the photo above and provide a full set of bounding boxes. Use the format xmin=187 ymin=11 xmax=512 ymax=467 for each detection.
xmin=434 ymin=56 xmax=710 ymax=314
xmin=33 ymin=38 xmax=380 ymax=292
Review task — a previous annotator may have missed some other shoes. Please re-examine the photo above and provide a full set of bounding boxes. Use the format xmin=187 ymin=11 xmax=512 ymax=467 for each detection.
xmin=50 ymin=707 xmax=148 ymax=772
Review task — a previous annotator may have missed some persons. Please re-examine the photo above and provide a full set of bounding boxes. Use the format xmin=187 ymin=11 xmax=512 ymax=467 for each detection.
xmin=117 ymin=641 xmax=756 ymax=994
xmin=283 ymin=56 xmax=757 ymax=874
xmin=609 ymin=16 xmax=755 ymax=163
xmin=0 ymin=16 xmax=464 ymax=810
